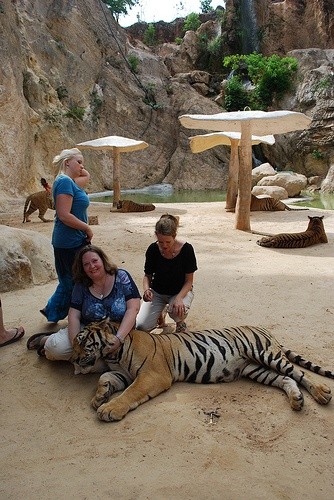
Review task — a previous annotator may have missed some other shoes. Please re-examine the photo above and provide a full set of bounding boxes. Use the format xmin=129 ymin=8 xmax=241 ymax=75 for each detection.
xmin=40 ymin=309 xmax=58 ymax=323
xmin=176 ymin=321 xmax=187 ymax=333
xmin=155 ymin=304 xmax=170 ymax=327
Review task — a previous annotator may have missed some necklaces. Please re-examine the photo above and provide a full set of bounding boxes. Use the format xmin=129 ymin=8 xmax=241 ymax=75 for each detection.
xmin=161 ymin=246 xmax=176 ymax=258
xmin=92 ymin=279 xmax=109 ymax=300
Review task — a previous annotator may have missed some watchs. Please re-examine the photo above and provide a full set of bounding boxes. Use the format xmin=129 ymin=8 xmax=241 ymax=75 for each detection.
xmin=116 ymin=334 xmax=125 ymax=345
xmin=143 ymin=288 xmax=153 ymax=292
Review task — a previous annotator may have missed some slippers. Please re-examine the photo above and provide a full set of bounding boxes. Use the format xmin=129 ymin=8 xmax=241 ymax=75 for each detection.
xmin=37 ymin=345 xmax=46 ymax=357
xmin=0 ymin=327 xmax=25 ymax=347
xmin=27 ymin=331 xmax=57 ymax=350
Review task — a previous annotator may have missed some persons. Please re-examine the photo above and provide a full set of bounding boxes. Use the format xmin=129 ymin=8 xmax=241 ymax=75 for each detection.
xmin=134 ymin=213 xmax=195 ymax=335
xmin=0 ymin=296 xmax=26 ymax=347
xmin=41 ymin=147 xmax=95 ymax=325
xmin=26 ymin=245 xmax=142 ymax=367
